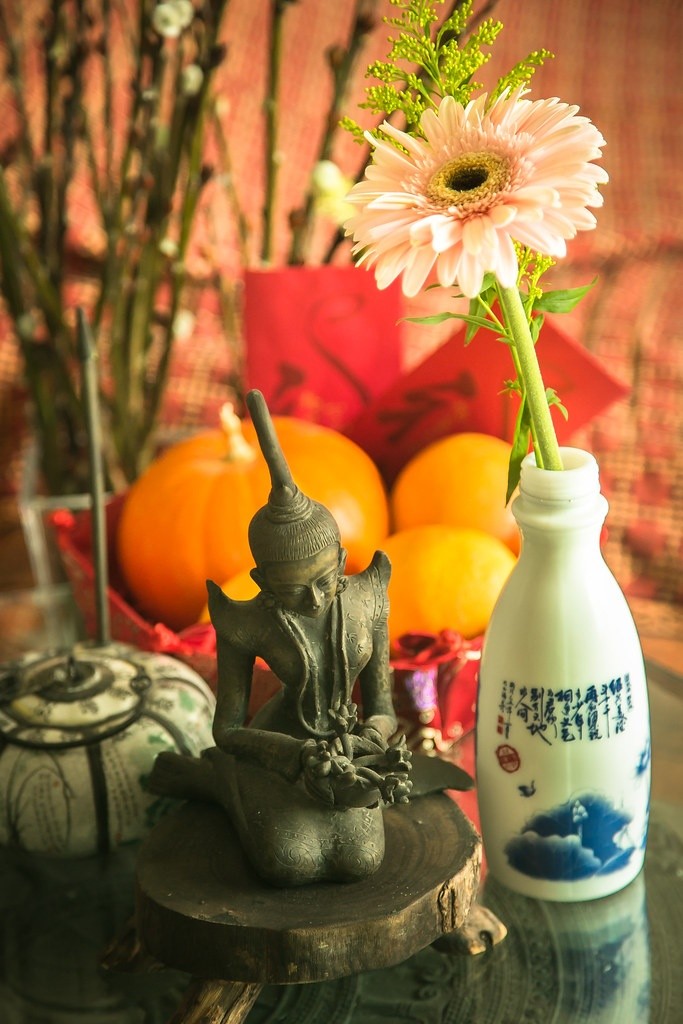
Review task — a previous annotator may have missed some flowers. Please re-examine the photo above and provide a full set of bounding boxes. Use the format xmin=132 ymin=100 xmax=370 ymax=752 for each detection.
xmin=330 ymin=0 xmax=609 ymax=505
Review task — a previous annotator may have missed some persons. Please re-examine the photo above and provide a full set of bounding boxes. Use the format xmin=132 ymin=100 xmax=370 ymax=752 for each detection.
xmin=136 ymin=385 xmax=399 ymax=889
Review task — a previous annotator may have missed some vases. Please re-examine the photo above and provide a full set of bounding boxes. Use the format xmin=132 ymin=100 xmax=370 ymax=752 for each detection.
xmin=478 ymin=448 xmax=650 ymax=903
